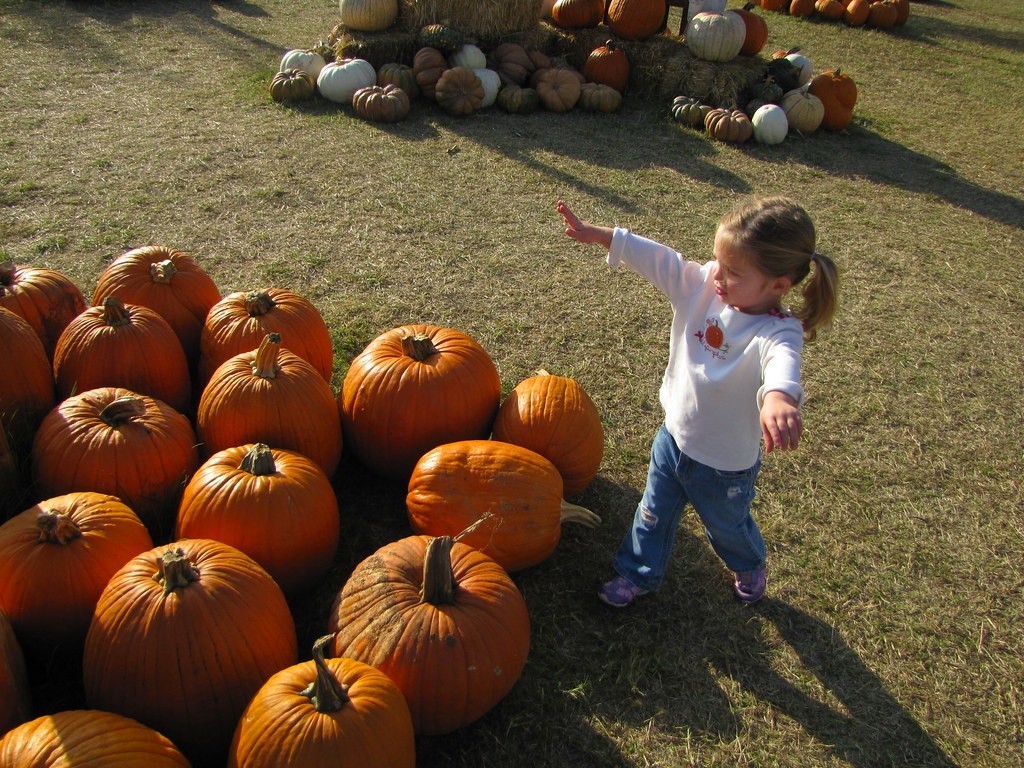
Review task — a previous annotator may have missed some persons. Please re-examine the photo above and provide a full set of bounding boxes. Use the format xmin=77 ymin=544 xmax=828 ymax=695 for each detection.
xmin=557 ymin=195 xmax=839 ymax=607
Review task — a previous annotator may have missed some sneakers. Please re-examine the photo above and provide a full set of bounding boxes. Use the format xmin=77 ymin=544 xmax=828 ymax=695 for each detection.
xmin=734 ymin=566 xmax=767 ymax=603
xmin=597 ymin=575 xmax=650 ymax=608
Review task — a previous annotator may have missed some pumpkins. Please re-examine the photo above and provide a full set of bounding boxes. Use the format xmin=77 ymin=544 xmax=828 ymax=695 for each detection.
xmin=0 ymin=246 xmax=605 ymax=768
xmin=267 ymin=0 xmax=857 ymax=144
xmin=750 ymin=0 xmax=910 ymax=30
